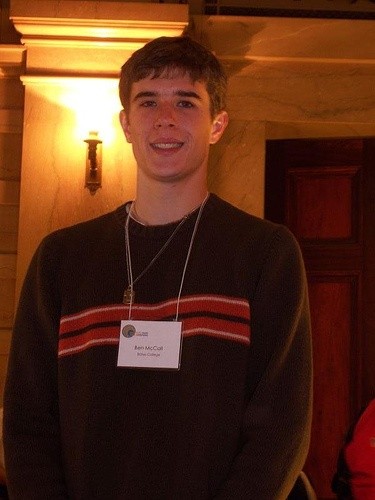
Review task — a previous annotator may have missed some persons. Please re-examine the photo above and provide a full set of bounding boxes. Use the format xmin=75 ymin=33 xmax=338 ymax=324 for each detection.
xmin=1 ymin=36 xmax=315 ymax=500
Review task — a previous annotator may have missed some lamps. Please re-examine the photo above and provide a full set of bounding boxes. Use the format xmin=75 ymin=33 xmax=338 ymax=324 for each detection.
xmin=82 ymin=103 xmax=103 ymax=196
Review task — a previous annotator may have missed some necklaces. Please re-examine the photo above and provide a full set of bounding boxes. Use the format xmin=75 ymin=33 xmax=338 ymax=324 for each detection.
xmin=123 ymin=195 xmax=206 ymax=307
